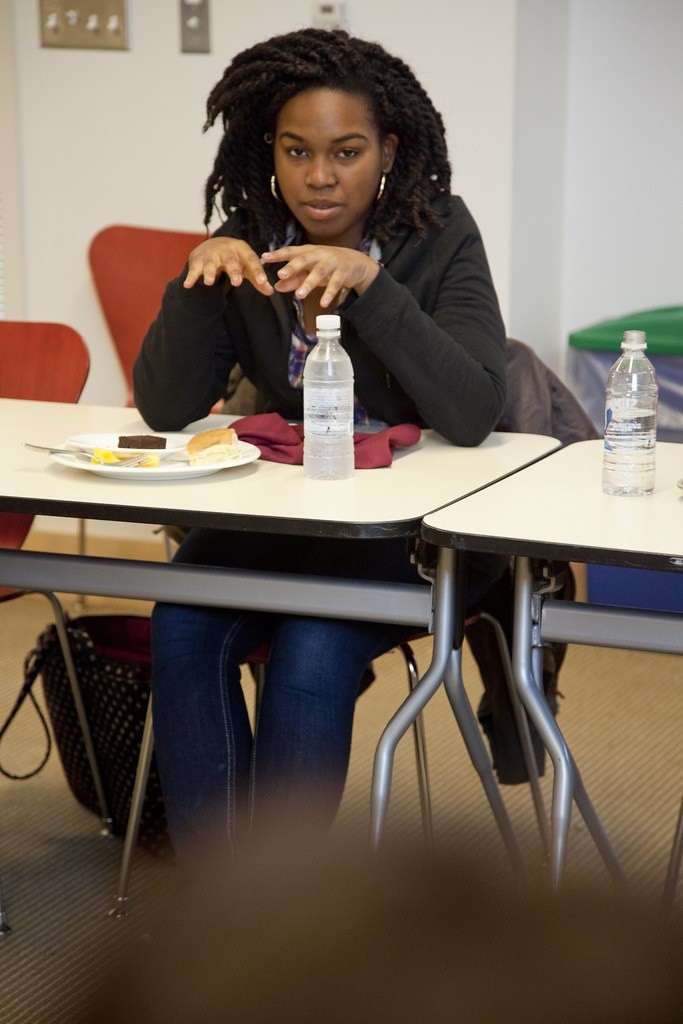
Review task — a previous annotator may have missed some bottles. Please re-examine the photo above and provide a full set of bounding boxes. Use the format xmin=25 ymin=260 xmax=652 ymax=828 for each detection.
xmin=302 ymin=315 xmax=355 ymax=478
xmin=601 ymin=331 xmax=659 ymax=496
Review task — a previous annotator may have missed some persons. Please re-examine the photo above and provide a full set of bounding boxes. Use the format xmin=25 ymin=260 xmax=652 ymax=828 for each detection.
xmin=128 ymin=26 xmax=509 ymax=901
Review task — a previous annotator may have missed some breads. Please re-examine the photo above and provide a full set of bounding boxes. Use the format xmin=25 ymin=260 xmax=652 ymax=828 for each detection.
xmin=186 ymin=427 xmax=240 ymax=466
xmin=118 ymin=435 xmax=166 ymax=449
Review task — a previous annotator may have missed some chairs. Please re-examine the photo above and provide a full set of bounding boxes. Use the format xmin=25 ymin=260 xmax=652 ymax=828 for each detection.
xmin=0 ymin=320 xmax=115 ymax=839
xmin=88 ymin=224 xmax=600 ymax=924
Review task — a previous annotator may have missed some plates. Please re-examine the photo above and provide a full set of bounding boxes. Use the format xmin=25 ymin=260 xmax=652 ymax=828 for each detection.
xmin=54 ymin=432 xmax=262 ymax=479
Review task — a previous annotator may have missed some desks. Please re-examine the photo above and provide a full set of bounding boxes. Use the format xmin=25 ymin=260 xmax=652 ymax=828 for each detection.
xmin=0 ymin=397 xmax=683 ymax=924
xmin=565 ymin=305 xmax=683 ymax=442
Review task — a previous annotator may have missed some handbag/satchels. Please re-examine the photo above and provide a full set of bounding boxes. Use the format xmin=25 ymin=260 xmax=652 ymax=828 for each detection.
xmin=0 ymin=613 xmax=156 ymax=853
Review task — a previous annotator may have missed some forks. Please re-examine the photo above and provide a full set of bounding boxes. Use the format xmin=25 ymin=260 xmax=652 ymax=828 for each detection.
xmin=22 ymin=441 xmax=149 ymax=469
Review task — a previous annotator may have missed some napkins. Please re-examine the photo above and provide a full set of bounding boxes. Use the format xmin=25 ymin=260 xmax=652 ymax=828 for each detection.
xmin=228 ymin=411 xmax=422 ymax=470
